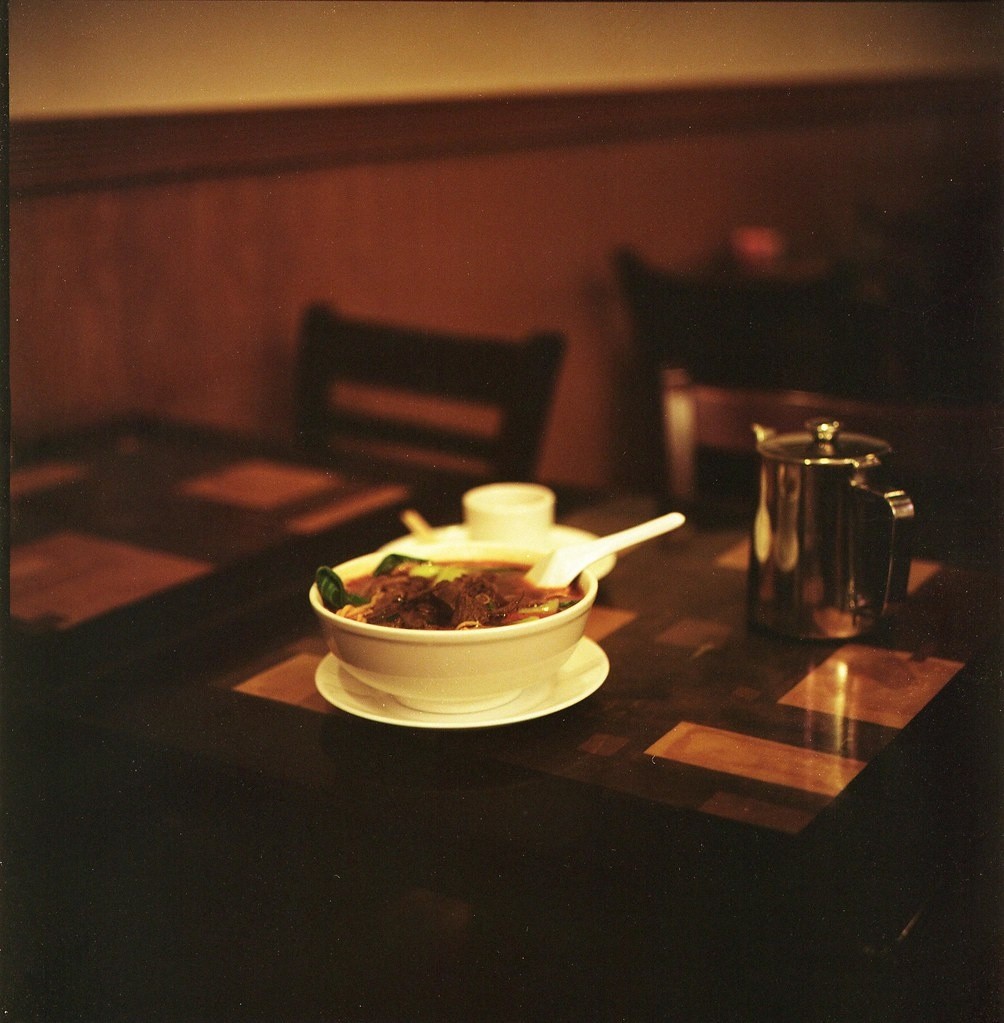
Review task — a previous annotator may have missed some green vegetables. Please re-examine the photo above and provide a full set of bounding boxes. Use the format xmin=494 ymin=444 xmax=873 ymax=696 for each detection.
xmin=315 ymin=554 xmax=580 ymax=628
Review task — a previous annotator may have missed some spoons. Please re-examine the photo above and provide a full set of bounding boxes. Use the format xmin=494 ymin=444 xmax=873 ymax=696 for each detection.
xmin=524 ymin=511 xmax=686 ymax=588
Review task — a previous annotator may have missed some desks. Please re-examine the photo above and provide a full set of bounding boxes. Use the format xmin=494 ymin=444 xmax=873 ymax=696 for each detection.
xmin=7 ymin=409 xmax=1004 ymax=1023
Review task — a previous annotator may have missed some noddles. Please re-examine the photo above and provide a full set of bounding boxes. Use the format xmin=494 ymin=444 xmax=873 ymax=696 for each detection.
xmin=330 ymin=562 xmax=573 ymax=631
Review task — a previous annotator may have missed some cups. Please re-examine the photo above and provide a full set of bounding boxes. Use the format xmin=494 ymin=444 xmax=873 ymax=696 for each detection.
xmin=461 ymin=482 xmax=555 ymax=524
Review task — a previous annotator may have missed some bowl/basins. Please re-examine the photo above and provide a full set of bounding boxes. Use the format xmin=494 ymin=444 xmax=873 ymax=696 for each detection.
xmin=308 ymin=543 xmax=599 ymax=715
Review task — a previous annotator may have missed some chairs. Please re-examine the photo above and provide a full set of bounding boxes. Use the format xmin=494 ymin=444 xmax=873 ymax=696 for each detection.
xmin=298 ymin=301 xmax=568 ymax=482
xmin=607 ymin=238 xmax=1004 ymax=575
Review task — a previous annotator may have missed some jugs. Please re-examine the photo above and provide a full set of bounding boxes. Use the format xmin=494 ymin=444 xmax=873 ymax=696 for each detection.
xmin=744 ymin=417 xmax=916 ymax=645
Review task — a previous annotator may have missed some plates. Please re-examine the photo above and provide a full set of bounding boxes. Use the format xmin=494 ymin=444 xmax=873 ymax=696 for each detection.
xmin=315 ymin=635 xmax=609 ymax=729
xmin=376 ymin=524 xmax=617 ymax=579
